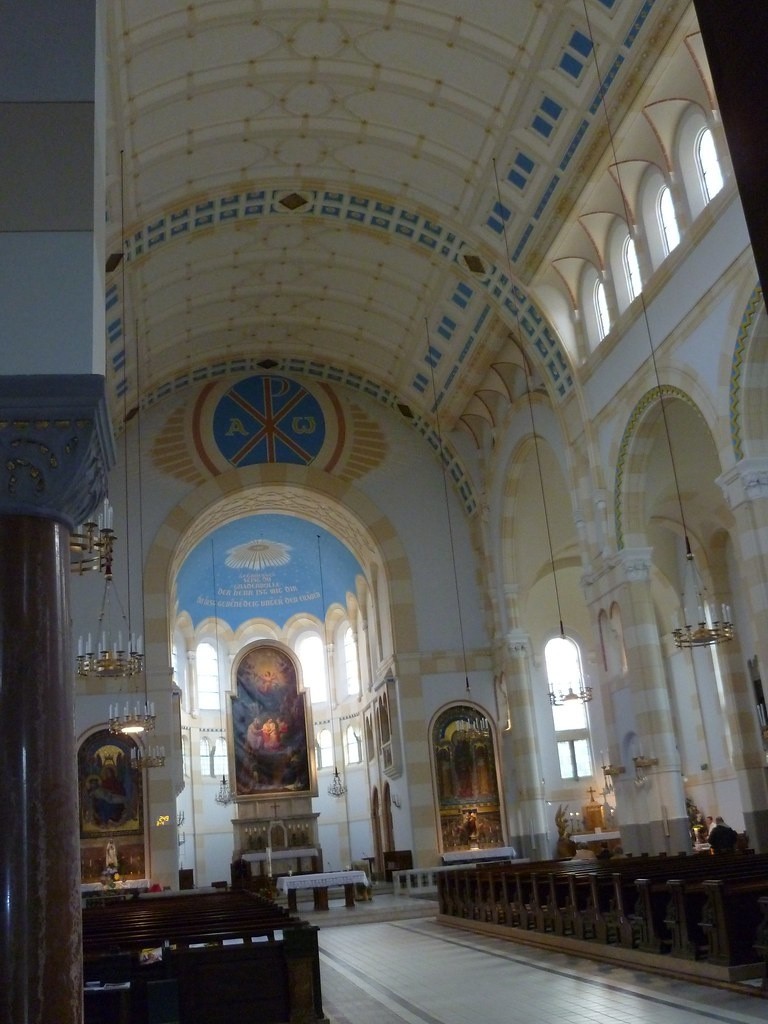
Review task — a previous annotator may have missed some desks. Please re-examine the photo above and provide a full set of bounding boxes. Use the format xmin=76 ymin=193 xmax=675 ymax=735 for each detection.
xmin=276 ymin=871 xmax=369 ymax=914
xmin=81 ymin=878 xmax=152 ymax=898
xmin=439 ymin=847 xmax=517 ymax=864
xmin=570 ymin=831 xmax=621 ymax=843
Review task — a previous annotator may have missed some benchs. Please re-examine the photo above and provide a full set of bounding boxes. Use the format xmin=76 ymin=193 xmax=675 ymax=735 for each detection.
xmin=82 ymin=886 xmax=331 ymax=1024
xmin=393 ymin=857 xmax=530 ymax=896
xmin=438 ymin=850 xmax=768 ymax=982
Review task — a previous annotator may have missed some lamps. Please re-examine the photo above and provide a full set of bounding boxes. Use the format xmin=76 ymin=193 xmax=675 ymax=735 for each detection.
xmin=599 ymin=751 xmax=626 ymax=794
xmin=392 ymin=794 xmax=400 ymax=809
xmin=75 ymin=565 xmax=145 ymax=679
xmin=215 ymin=775 xmax=237 ymax=807
xmin=245 ymin=826 xmax=266 ymax=850
xmin=177 ymin=811 xmax=184 ymax=827
xmin=328 ymin=767 xmax=347 ymax=800
xmin=109 ymin=674 xmax=156 ymax=736
xmin=671 ymin=536 xmax=733 ymax=651
xmin=289 ymin=823 xmax=309 ymax=845
xmin=130 ymin=745 xmax=167 ymax=770
xmin=457 ymin=677 xmax=490 ymax=744
xmin=631 ymin=743 xmax=659 ymax=788
xmin=71 ymin=497 xmax=116 ymax=577
xmin=548 ymin=682 xmax=593 ymax=707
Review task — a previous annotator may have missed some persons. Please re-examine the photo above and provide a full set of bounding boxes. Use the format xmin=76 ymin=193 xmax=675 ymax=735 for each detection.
xmin=557 ymin=831 xmax=627 ymax=861
xmin=106 ymin=839 xmax=118 ymax=867
xmin=465 ymin=810 xmax=477 ymax=838
xmin=706 ymin=816 xmax=737 ymax=854
xmin=557 ymin=818 xmax=569 ymax=838
